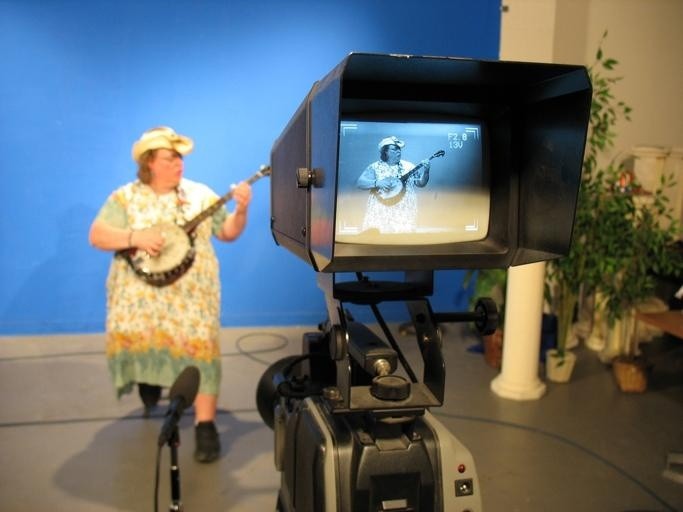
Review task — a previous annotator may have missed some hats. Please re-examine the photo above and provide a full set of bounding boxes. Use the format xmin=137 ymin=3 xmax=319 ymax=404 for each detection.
xmin=133 ymin=128 xmax=192 ymax=160
xmin=378 ymin=137 xmax=404 ymax=148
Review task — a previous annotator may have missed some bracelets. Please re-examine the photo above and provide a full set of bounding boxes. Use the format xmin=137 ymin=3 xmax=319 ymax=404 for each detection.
xmin=126 ymin=230 xmax=135 ymax=248
xmin=373 ymin=180 xmax=376 ymax=189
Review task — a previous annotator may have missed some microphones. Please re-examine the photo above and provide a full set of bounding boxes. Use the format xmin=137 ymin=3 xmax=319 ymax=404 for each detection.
xmin=157 ymin=365 xmax=200 ymax=445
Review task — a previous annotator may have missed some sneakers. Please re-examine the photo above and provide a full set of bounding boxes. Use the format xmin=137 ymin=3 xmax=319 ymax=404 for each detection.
xmin=140 ymin=383 xmax=162 ymax=406
xmin=196 ymin=422 xmax=220 ymax=463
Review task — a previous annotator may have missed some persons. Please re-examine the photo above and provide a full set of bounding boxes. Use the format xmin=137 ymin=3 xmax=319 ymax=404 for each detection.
xmin=350 ymin=134 xmax=429 ymax=232
xmin=87 ymin=124 xmax=253 ymax=464
